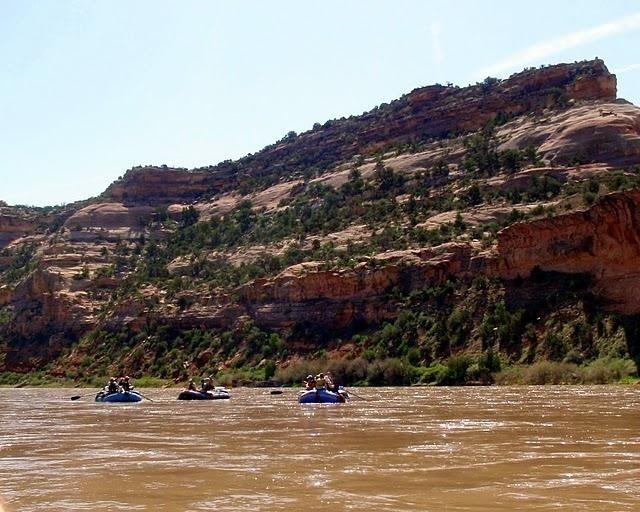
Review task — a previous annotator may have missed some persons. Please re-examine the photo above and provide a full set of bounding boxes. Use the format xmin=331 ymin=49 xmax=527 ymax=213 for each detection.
xmin=189 ymin=376 xmax=215 ymax=393
xmin=304 ymin=371 xmax=337 ymax=392
xmin=101 ymin=376 xmax=134 ymax=394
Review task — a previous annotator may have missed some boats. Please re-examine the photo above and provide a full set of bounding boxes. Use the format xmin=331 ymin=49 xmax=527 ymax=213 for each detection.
xmin=297 ymin=385 xmax=350 ymax=404
xmin=175 ymin=389 xmax=232 ymax=401
xmin=92 ymin=392 xmax=142 ymax=403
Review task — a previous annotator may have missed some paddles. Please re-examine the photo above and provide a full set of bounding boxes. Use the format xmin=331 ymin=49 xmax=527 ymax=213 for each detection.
xmin=71 ymin=391 xmax=99 ymax=400
